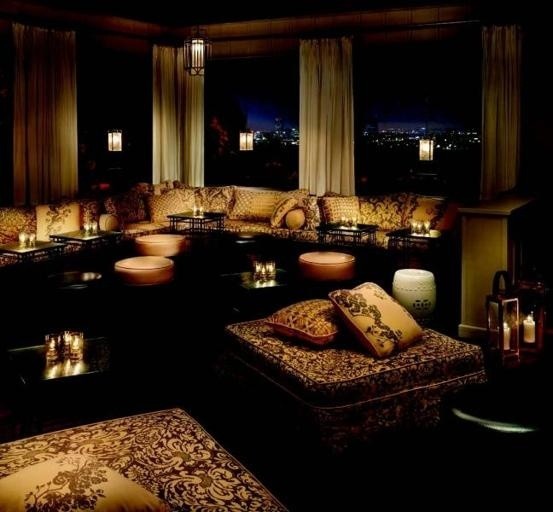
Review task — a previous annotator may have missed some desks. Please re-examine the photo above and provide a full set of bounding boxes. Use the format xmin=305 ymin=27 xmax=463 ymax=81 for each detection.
xmin=169 ymin=210 xmax=222 ymax=240
xmin=317 ymin=221 xmax=379 ymax=249
xmin=49 ymin=222 xmax=123 ymax=268
xmin=384 ymin=228 xmax=444 ymax=263
xmin=0 ymin=235 xmax=66 ymax=277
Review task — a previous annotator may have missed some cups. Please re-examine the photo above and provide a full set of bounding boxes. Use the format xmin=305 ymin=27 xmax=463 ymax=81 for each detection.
xmin=193 ymin=206 xmax=203 ymax=216
xmin=339 ymin=213 xmax=358 ymax=231
xmin=17 ymin=232 xmax=35 ymax=246
xmin=253 ymin=261 xmax=276 ymax=281
xmin=41 ymin=331 xmax=84 ymax=364
xmin=410 ymin=219 xmax=431 ymax=237
xmin=82 ymin=220 xmax=97 ymax=236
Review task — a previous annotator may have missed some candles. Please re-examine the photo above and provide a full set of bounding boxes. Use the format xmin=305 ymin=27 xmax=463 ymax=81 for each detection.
xmin=521 ymin=314 xmax=534 ymax=344
xmin=499 ymin=322 xmax=511 ymax=351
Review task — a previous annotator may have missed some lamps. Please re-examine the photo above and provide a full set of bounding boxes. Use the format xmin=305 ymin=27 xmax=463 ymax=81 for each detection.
xmin=105 ymin=122 xmax=123 ymax=153
xmin=183 ymin=3 xmax=212 ymax=76
xmin=239 ymin=122 xmax=253 ymax=151
xmin=418 ymin=133 xmax=434 ymax=162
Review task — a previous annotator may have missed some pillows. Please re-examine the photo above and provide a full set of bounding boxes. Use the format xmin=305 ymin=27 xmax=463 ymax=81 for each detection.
xmin=146 ymin=195 xmax=187 ymax=223
xmin=268 ymin=194 xmax=300 ymax=227
xmin=101 ymin=191 xmax=147 ymax=222
xmin=0 ymin=455 xmax=161 ymax=512
xmin=231 ymin=188 xmax=297 ymax=219
xmin=266 ymin=294 xmax=345 ymax=345
xmin=321 ymin=195 xmax=363 ymax=228
xmin=328 ymin=282 xmax=423 ymax=356
xmin=172 ymin=179 xmax=236 ymax=218
xmin=403 ymin=192 xmax=450 ymax=231
xmin=33 ymin=204 xmax=81 ymax=242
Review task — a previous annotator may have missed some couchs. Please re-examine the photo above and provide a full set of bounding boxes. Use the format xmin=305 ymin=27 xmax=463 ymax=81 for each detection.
xmin=0 ymin=181 xmax=462 ymax=267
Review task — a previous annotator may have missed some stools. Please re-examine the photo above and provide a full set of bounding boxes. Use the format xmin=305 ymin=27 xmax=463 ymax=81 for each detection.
xmin=1 ymin=406 xmax=288 ymax=511
xmin=393 ymin=268 xmax=434 ymax=320
xmin=298 ymin=251 xmax=356 ymax=281
xmin=136 ymin=234 xmax=189 ymax=258
xmin=114 ymin=256 xmax=176 ymax=288
xmin=223 ymin=320 xmax=488 ymax=447
